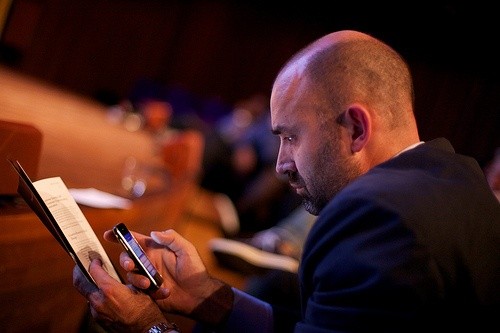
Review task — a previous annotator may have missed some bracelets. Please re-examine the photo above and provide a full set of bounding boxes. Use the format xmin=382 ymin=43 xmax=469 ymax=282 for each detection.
xmin=143 ymin=322 xmax=180 ymax=333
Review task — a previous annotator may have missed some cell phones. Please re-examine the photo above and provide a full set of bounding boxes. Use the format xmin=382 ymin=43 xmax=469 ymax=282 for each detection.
xmin=113 ymin=223 xmax=164 ymax=292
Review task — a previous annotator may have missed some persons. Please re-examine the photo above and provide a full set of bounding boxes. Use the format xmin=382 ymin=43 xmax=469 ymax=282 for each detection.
xmin=72 ymin=28 xmax=500 ymax=333
